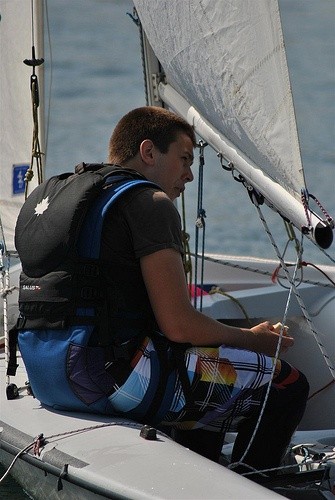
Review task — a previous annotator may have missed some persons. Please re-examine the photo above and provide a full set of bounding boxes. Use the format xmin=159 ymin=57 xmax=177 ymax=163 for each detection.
xmin=16 ymin=106 xmax=311 ymax=479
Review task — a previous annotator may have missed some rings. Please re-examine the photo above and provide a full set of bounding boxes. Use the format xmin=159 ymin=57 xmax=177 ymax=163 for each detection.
xmin=286 ymin=346 xmax=288 ymax=352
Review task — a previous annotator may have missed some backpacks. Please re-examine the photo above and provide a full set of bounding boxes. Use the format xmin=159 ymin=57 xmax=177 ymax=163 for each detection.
xmin=15 ymin=163 xmax=164 ymax=418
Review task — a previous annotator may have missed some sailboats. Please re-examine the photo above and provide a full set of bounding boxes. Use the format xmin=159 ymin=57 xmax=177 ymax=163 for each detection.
xmin=0 ymin=0 xmax=335 ymax=499
